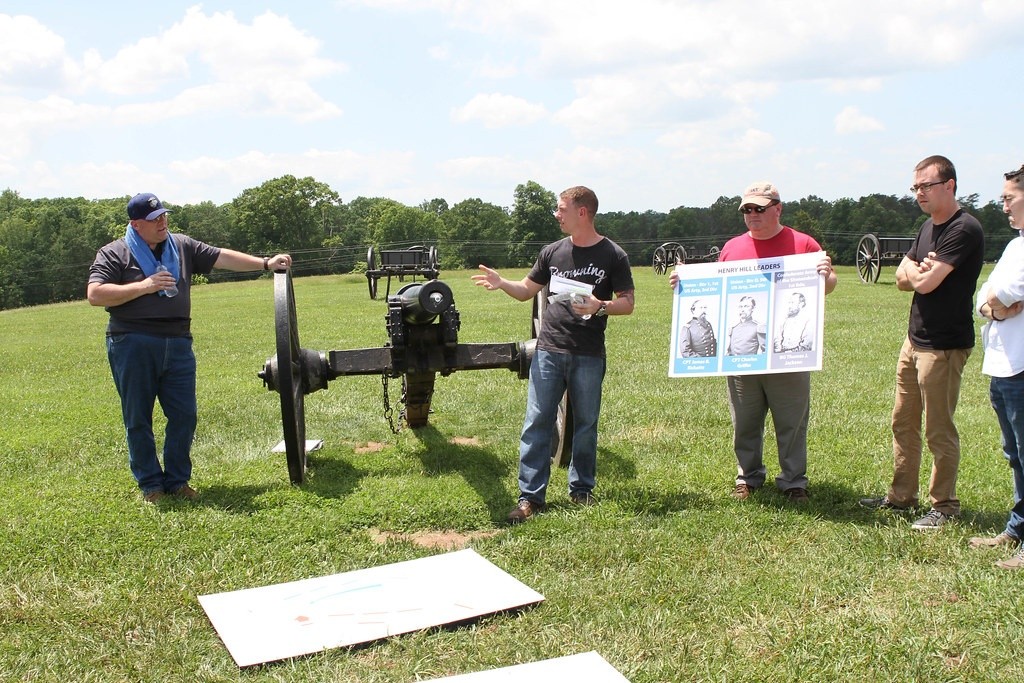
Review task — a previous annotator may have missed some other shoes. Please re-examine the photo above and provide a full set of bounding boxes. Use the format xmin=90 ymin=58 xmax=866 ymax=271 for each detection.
xmin=172 ymin=486 xmax=198 ymax=499
xmin=144 ymin=491 xmax=165 ymax=505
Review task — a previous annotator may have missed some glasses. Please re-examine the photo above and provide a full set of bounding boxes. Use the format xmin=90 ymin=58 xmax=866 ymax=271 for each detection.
xmin=910 ymin=181 xmax=947 ymax=194
xmin=743 ymin=201 xmax=780 ymax=214
xmin=135 ymin=211 xmax=170 ymax=224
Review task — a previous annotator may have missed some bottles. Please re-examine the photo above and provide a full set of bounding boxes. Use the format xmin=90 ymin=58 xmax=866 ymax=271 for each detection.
xmin=569 ymin=293 xmax=591 ymax=320
xmin=156 ymin=261 xmax=178 ymax=297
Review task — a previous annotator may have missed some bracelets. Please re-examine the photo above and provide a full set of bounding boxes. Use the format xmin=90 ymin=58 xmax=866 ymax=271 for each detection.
xmin=992 ymin=309 xmax=1005 ymax=321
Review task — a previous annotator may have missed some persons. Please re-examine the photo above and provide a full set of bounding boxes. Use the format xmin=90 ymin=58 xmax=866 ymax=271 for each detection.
xmin=669 ymin=182 xmax=837 ymax=503
xmin=471 ymin=186 xmax=635 ymax=522
xmin=975 ymin=164 xmax=1024 ymax=569
xmin=87 ymin=193 xmax=292 ymax=504
xmin=858 ymin=155 xmax=985 ymax=530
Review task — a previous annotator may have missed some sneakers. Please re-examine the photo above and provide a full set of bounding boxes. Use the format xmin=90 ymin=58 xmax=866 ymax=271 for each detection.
xmin=911 ymin=508 xmax=951 ymax=530
xmin=785 ymin=487 xmax=810 ymax=504
xmin=993 ymin=550 xmax=1024 ymax=572
xmin=971 ymin=531 xmax=1015 ymax=547
xmin=506 ymin=499 xmax=537 ymax=521
xmin=730 ymin=484 xmax=755 ymax=500
xmin=570 ymin=493 xmax=592 ymax=509
xmin=858 ymin=496 xmax=906 ymax=512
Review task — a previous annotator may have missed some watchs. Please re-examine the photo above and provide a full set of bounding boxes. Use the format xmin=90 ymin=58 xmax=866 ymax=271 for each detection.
xmin=592 ymin=301 xmax=606 ymax=318
xmin=263 ymin=257 xmax=270 ymax=270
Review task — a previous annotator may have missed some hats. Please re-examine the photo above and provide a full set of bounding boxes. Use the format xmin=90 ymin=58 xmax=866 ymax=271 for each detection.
xmin=738 ymin=181 xmax=780 ymax=211
xmin=126 ymin=193 xmax=172 ymax=221
